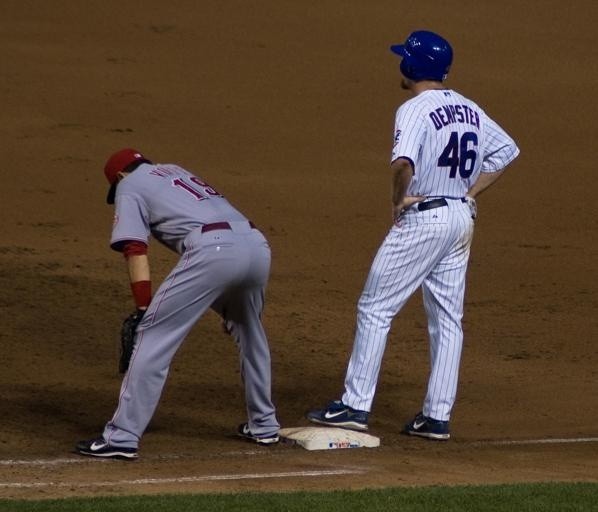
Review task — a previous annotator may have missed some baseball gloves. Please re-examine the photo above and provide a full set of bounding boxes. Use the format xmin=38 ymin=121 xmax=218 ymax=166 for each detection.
xmin=120 ymin=308 xmax=148 ymax=373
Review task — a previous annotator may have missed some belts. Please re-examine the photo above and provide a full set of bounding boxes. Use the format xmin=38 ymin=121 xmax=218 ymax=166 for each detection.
xmin=419 ymin=198 xmax=466 ymax=211
xmin=202 ymin=221 xmax=257 ymax=233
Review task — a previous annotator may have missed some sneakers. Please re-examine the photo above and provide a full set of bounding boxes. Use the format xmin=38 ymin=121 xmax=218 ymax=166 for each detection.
xmin=307 ymin=399 xmax=368 ymax=432
xmin=403 ymin=412 xmax=451 ymax=440
xmin=238 ymin=423 xmax=279 ymax=445
xmin=76 ymin=436 xmax=138 ymax=459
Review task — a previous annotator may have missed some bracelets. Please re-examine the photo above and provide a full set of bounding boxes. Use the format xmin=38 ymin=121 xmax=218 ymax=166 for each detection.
xmin=128 ymin=280 xmax=151 ymax=308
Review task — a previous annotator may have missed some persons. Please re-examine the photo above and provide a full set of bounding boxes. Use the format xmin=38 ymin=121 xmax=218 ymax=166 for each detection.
xmin=72 ymin=147 xmax=282 ymax=461
xmin=307 ymin=29 xmax=520 ymax=440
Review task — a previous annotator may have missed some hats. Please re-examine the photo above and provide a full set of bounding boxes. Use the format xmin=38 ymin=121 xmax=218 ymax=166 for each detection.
xmin=104 ymin=148 xmax=152 ymax=204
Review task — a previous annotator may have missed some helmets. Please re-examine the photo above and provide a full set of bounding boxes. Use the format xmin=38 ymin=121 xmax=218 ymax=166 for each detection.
xmin=390 ymin=30 xmax=453 ymax=82
